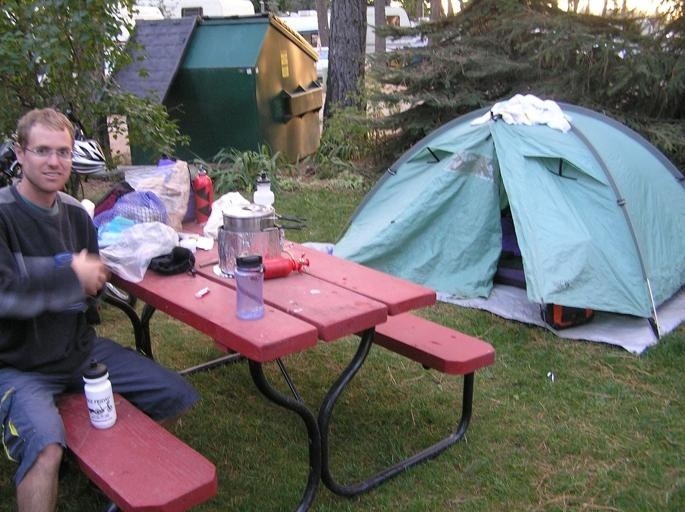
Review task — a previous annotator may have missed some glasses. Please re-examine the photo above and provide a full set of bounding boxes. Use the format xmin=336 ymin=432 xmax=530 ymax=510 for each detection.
xmin=21 ymin=145 xmax=71 ymax=159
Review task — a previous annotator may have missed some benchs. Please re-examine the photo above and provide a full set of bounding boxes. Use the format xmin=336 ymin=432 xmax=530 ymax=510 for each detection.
xmin=318 ymin=311 xmax=493 ymax=497
xmin=57 ymin=388 xmax=319 ymax=511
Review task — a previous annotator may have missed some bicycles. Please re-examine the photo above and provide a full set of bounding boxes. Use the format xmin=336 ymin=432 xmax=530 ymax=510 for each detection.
xmin=0 ymin=95 xmax=87 ymax=198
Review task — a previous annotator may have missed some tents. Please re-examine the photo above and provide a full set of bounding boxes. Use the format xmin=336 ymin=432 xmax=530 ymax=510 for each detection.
xmin=331 ymin=95 xmax=685 ymax=356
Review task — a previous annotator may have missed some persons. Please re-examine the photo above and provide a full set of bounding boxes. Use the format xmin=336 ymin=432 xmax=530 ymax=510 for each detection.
xmin=0 ymin=107 xmax=197 ymax=510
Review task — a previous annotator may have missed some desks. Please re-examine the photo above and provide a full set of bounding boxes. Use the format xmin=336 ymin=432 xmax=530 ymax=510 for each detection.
xmin=110 ymin=230 xmax=436 ymax=511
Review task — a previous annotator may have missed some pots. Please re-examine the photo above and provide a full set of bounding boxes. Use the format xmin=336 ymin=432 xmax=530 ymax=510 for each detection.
xmin=215 ymin=201 xmax=309 ymax=276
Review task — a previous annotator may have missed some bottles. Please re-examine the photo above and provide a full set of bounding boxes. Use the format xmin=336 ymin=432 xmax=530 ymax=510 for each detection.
xmin=253 ymin=171 xmax=275 ymax=206
xmin=263 ymin=254 xmax=310 ymax=279
xmin=192 ymin=163 xmax=214 ymax=225
xmin=81 ymin=357 xmax=118 ymax=431
xmin=233 ymin=253 xmax=263 ymax=321
